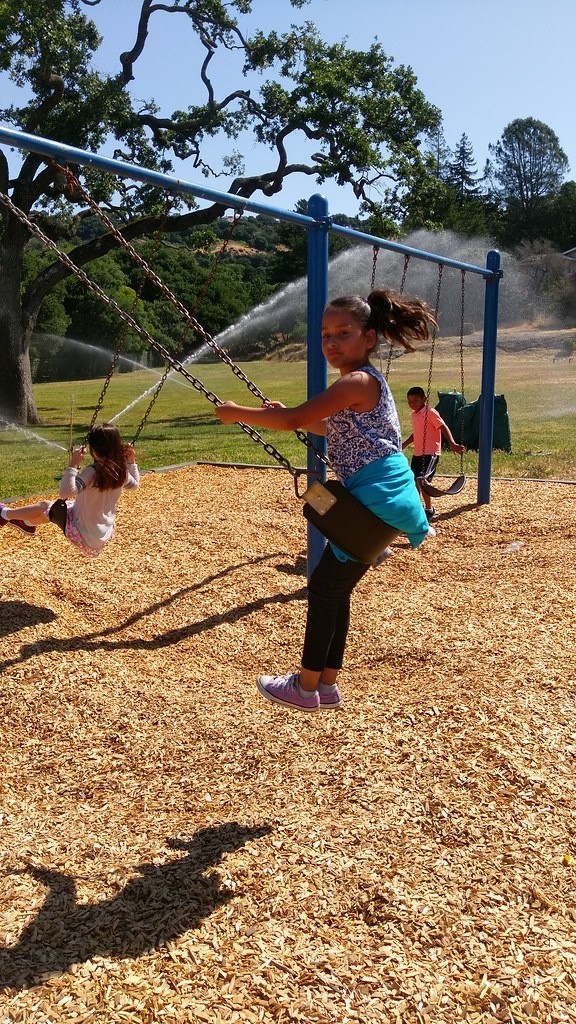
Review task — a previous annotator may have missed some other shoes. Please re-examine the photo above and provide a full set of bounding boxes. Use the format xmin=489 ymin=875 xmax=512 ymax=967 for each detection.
xmin=424 ymin=506 xmax=435 ymax=521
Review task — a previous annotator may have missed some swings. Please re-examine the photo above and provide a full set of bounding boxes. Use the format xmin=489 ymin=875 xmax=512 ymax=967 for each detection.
xmin=47 ymin=191 xmax=244 ymax=539
xmin=0 ymin=159 xmax=412 ymax=570
xmin=415 ymin=262 xmax=468 ymax=499
xmin=370 ymin=245 xmax=411 ymax=382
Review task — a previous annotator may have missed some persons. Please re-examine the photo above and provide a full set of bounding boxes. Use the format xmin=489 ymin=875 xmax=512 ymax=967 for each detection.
xmin=0 ymin=424 xmax=140 ymax=557
xmin=402 ymin=387 xmax=467 ymax=520
xmin=215 ymin=289 xmax=439 ymax=711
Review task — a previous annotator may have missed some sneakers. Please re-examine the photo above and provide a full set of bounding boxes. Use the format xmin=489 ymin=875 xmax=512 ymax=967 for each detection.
xmin=0 ymin=503 xmax=8 ymax=526
xmin=256 ymin=674 xmax=320 ymax=712
xmin=10 ymin=519 xmax=37 ymax=534
xmin=319 ymin=689 xmax=343 ymax=709
